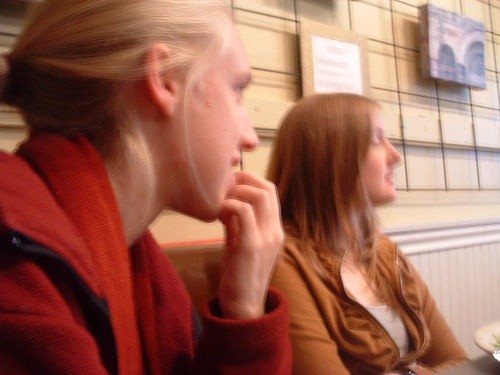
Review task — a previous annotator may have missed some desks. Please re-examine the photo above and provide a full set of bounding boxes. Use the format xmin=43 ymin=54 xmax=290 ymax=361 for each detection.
xmin=475 ymin=324 xmax=500 ymax=356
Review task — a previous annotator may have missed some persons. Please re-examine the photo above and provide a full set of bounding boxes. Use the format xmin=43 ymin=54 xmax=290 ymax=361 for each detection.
xmin=0 ymin=0 xmax=292 ymax=375
xmin=267 ymin=93 xmax=500 ymax=375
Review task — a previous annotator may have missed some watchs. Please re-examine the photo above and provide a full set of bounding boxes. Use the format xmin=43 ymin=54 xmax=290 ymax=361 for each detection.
xmin=398 ymin=364 xmax=417 ymax=375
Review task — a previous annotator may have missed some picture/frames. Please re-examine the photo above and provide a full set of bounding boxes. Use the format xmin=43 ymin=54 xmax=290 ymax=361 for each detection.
xmin=299 ymin=17 xmax=370 ymax=100
